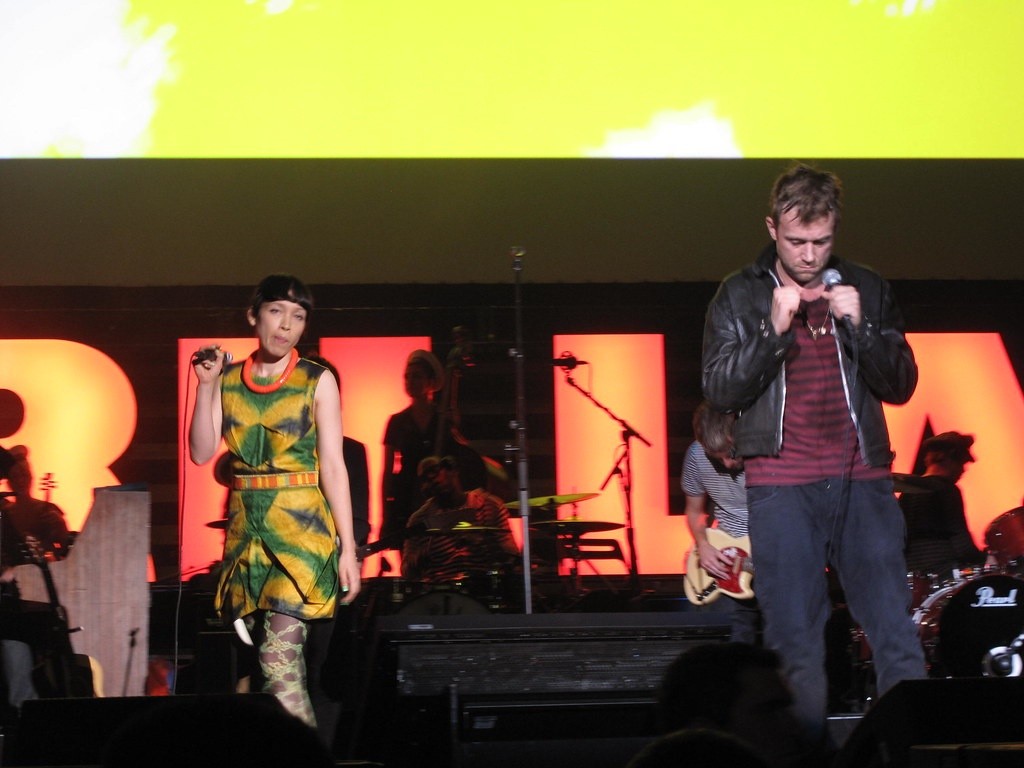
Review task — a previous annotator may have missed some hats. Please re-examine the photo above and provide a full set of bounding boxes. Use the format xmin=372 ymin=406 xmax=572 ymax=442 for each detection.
xmin=922 ymin=431 xmax=975 ymax=465
xmin=410 ymin=349 xmax=444 ymax=393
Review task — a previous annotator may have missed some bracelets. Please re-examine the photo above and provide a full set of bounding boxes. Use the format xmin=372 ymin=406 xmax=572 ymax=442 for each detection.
xmin=341 ymin=552 xmax=358 ymax=559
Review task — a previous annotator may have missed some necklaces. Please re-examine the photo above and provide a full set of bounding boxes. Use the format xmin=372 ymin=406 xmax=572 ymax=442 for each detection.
xmin=801 ymin=307 xmax=832 ymax=340
xmin=241 ymin=347 xmax=298 ymax=394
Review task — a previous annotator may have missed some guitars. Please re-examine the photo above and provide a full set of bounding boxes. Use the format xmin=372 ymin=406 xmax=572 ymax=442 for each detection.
xmin=683 ymin=527 xmax=757 ymax=606
xmin=356 ymin=507 xmax=480 ymax=560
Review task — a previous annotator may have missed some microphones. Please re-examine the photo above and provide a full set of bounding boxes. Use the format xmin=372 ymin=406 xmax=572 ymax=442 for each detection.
xmin=990 ymin=633 xmax=1024 ymax=676
xmin=195 ymin=349 xmax=233 ymax=363
xmin=553 ymin=359 xmax=588 ymax=366
xmin=822 ymin=269 xmax=856 ymax=330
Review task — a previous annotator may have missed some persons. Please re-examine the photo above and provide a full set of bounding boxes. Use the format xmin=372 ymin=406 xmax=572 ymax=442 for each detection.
xmin=628 ymin=572 xmax=1024 ymax=768
xmin=298 ymin=351 xmax=371 ymax=576
xmin=0 ymin=445 xmax=67 ymax=536
xmin=400 ymin=445 xmax=520 ymax=589
xmin=188 ymin=274 xmax=361 ymax=728
xmin=898 ymin=431 xmax=988 ymax=574
xmin=678 ymin=401 xmax=760 ymax=651
xmin=699 ymin=164 xmax=930 ymax=748
xmin=379 ymin=349 xmax=455 ymax=559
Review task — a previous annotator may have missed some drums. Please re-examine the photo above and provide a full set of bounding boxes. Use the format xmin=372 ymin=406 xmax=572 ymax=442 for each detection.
xmin=906 ymin=572 xmax=946 ymax=615
xmin=849 ymin=624 xmax=874 ymax=668
xmin=913 ymin=572 xmax=1024 ymax=680
xmin=381 ymin=582 xmax=489 ymax=615
xmin=985 ymin=506 xmax=1024 ymax=574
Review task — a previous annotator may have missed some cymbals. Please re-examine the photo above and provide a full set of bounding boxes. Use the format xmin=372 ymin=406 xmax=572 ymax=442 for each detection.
xmin=529 ymin=517 xmax=629 ymax=534
xmin=891 ymin=471 xmax=944 ymax=494
xmin=502 ymin=492 xmax=601 ymax=510
xmin=423 ymin=526 xmax=512 ymax=535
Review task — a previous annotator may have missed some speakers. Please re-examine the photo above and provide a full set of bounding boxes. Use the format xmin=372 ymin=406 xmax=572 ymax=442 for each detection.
xmin=840 ymin=677 xmax=1023 ymax=768
xmin=375 ymin=622 xmax=733 ymax=768
xmin=14 ymin=694 xmax=338 ymax=768
